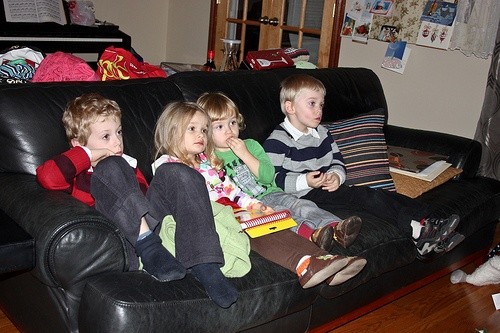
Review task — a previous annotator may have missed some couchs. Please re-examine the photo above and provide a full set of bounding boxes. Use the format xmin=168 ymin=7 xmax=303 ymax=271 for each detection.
xmin=0 ymin=67 xmax=500 ymax=333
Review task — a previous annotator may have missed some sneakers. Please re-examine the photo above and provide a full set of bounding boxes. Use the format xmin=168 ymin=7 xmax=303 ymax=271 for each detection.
xmin=430 ymin=232 xmax=464 ymax=256
xmin=411 ymin=215 xmax=460 ymax=255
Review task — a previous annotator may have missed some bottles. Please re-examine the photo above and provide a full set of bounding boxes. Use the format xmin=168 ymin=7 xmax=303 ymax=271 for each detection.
xmin=203 ymin=49 xmax=216 ymax=72
xmin=220 ymin=38 xmax=240 ymax=72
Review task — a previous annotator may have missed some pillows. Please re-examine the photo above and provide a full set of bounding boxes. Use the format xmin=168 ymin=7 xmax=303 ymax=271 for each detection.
xmin=322 ymin=113 xmax=397 ymax=193
xmin=380 ymin=165 xmax=462 ymax=199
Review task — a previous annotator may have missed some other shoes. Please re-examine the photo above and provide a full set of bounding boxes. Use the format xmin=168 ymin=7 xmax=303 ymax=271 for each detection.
xmin=309 ymin=225 xmax=335 ymax=251
xmin=334 ymin=216 xmax=362 ymax=248
xmin=296 ymin=254 xmax=348 ymax=288
xmin=326 ymin=256 xmax=366 ymax=285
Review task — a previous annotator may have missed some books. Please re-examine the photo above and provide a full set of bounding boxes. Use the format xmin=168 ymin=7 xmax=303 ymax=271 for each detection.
xmin=233 ymin=207 xmax=291 ymax=229
xmin=386 ymin=144 xmax=453 ymax=182
xmin=3 ymin=0 xmax=67 ymax=25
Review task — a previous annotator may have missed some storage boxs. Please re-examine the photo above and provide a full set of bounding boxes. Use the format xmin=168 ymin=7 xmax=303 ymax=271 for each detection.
xmin=246 ymin=48 xmax=294 ymax=71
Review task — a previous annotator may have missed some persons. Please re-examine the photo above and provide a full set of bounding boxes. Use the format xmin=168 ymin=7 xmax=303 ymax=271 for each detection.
xmin=197 ymin=92 xmax=362 ymax=251
xmin=263 ymin=75 xmax=466 ymax=254
xmin=35 ymin=94 xmax=239 ymax=308
xmin=149 ymin=101 xmax=367 ymax=289
